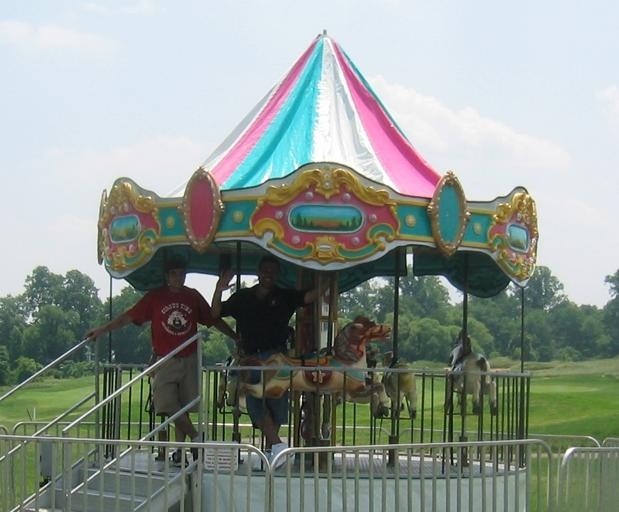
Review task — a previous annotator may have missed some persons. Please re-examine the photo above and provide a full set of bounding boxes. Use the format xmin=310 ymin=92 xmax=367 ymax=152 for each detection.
xmin=211 ymin=255 xmax=332 ymax=474
xmin=80 ymin=262 xmax=239 ymax=468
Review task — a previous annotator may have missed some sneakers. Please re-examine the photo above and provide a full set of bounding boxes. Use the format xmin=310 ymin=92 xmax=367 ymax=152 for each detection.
xmin=170 ymin=440 xmax=200 ymax=468
xmin=266 ymin=442 xmax=293 ymax=471
